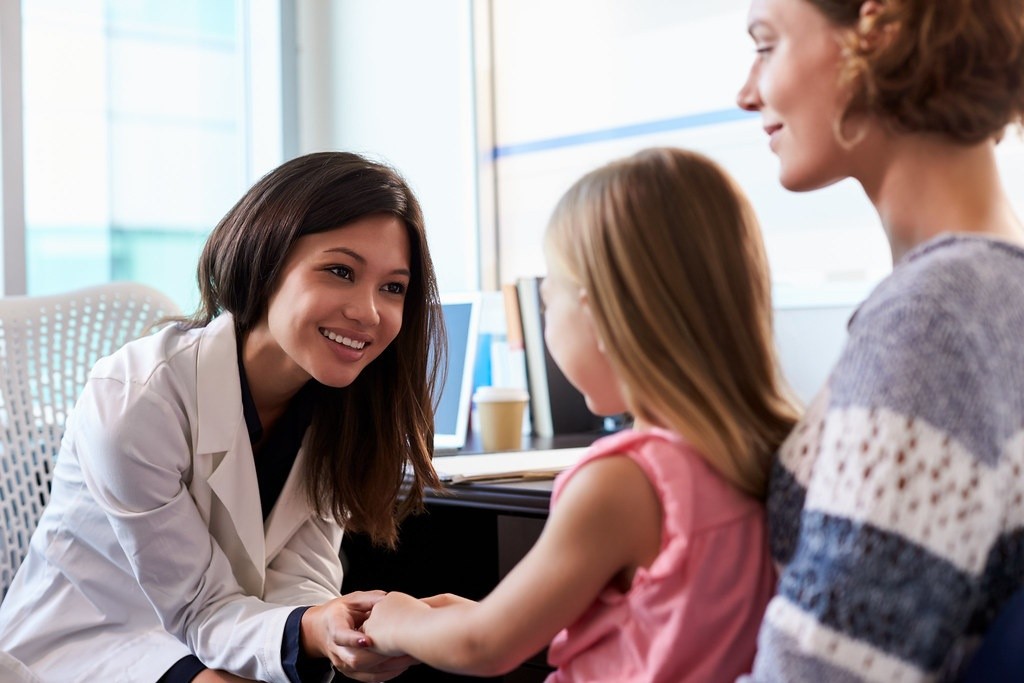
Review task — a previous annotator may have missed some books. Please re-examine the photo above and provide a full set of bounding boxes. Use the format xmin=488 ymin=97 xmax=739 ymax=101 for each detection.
xmin=499 ymin=276 xmax=606 ymax=439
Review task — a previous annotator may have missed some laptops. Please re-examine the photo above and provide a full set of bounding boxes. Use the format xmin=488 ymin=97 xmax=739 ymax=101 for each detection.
xmin=403 ymin=291 xmax=484 ymax=449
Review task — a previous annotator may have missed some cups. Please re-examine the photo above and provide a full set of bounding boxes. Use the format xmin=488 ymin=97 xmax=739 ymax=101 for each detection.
xmin=471 ymin=385 xmax=531 ymax=453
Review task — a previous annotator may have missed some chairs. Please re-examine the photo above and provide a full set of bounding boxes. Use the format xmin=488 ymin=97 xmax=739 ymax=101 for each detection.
xmin=0 ymin=282 xmax=186 ymax=600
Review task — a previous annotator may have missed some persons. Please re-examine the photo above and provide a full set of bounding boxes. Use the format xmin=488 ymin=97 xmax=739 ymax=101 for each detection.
xmin=0 ymin=148 xmax=443 ymax=683
xmin=732 ymin=0 xmax=1024 ymax=683
xmin=358 ymin=146 xmax=802 ymax=683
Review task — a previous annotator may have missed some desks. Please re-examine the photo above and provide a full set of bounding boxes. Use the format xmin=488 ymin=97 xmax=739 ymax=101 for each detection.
xmin=395 ymin=431 xmax=611 ymax=585
